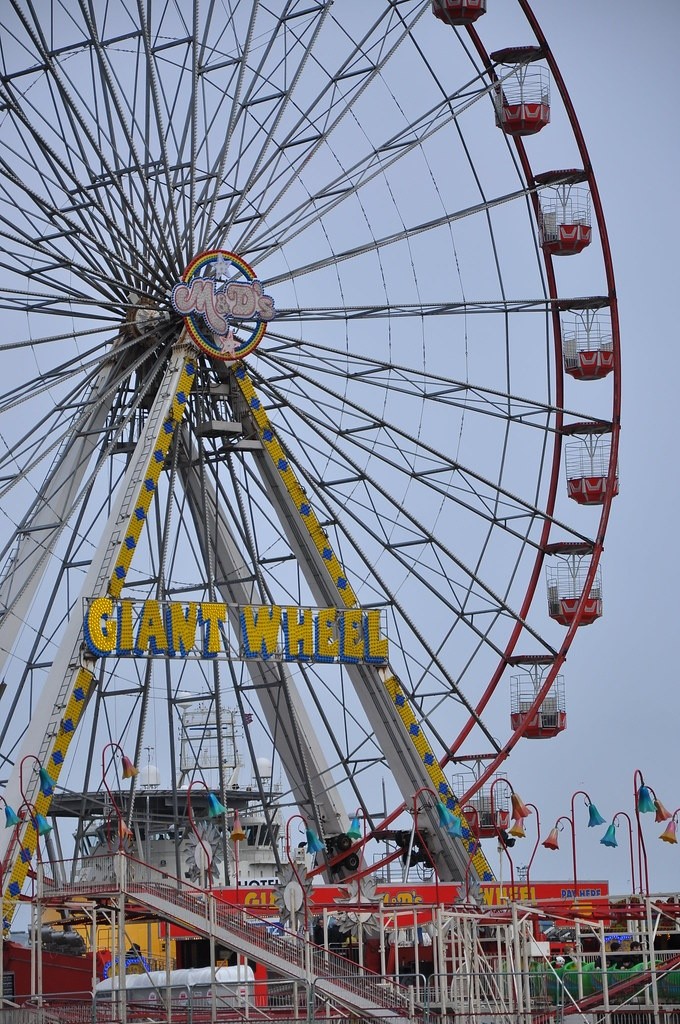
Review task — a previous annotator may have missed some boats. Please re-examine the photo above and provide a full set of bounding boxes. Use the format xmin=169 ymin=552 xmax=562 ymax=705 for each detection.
xmin=43 ymin=696 xmax=313 ymax=881
